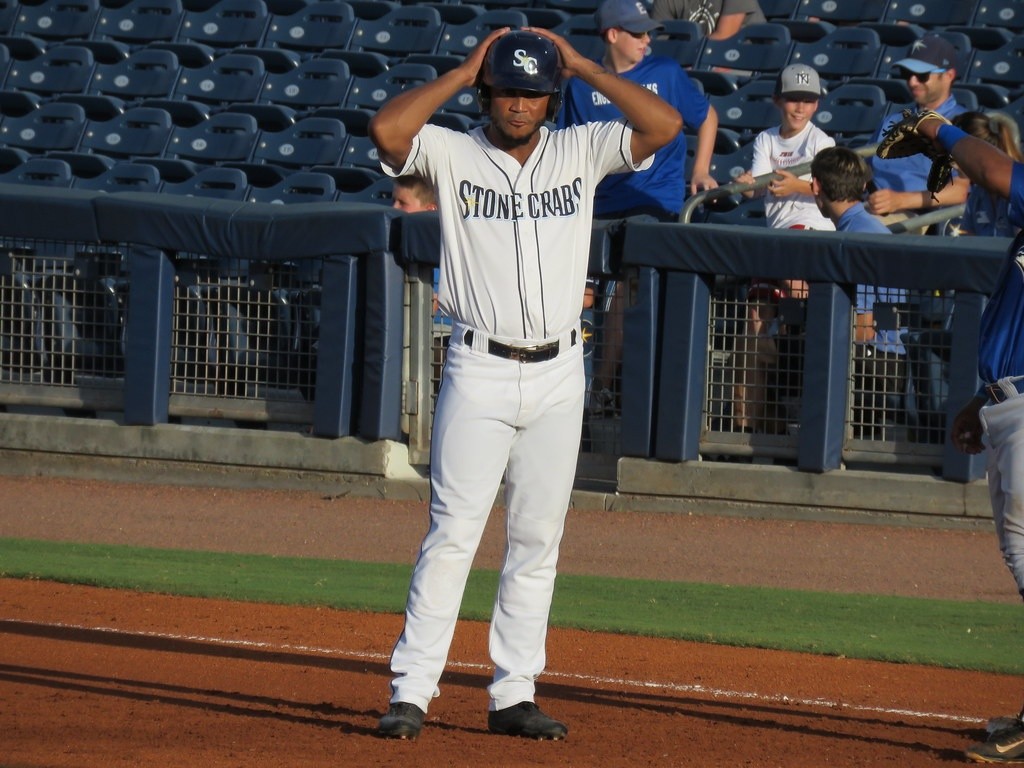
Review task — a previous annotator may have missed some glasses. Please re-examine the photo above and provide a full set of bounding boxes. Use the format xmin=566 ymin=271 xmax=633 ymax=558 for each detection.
xmin=627 ymin=30 xmax=650 ymax=38
xmin=899 ymin=66 xmax=946 ymax=83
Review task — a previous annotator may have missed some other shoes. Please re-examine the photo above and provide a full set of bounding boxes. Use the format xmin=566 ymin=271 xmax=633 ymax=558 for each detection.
xmin=590 ymin=386 xmax=616 ymax=418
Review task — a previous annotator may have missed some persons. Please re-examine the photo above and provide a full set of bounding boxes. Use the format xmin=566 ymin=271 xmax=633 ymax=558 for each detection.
xmin=652 ymin=0 xmax=767 ymax=153
xmin=370 ymin=28 xmax=684 ymax=741
xmin=758 ymin=147 xmax=907 ymax=467
xmin=950 ymin=112 xmax=1024 ymax=238
xmin=727 ymin=62 xmax=837 ymax=434
xmin=391 ymin=172 xmax=455 ymax=329
xmin=556 ymin=0 xmax=720 ymax=419
xmin=853 ymin=35 xmax=971 ymax=441
xmin=876 ymin=108 xmax=1024 ymax=764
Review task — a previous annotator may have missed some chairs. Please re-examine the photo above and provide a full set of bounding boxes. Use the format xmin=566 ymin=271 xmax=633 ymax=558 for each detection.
xmin=1 ymin=0 xmax=1024 ymax=446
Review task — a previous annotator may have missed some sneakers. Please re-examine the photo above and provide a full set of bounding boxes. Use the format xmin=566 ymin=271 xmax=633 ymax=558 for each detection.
xmin=488 ymin=701 xmax=568 ymax=741
xmin=965 ymin=719 xmax=1024 ymax=763
xmin=377 ymin=702 xmax=426 ymax=740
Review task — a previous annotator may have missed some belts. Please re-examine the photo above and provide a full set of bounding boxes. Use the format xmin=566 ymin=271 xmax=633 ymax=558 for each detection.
xmin=464 ymin=327 xmax=576 ymax=363
xmin=990 ymin=377 xmax=1024 ymax=406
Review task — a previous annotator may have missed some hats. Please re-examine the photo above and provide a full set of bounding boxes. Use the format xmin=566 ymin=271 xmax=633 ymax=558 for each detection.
xmin=891 ymin=34 xmax=955 ymax=74
xmin=593 ymin=0 xmax=665 ymax=33
xmin=774 ymin=63 xmax=821 ymax=99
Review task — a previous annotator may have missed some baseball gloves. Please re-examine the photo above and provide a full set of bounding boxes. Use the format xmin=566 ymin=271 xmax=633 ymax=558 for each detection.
xmin=875 ymin=108 xmax=955 ymax=194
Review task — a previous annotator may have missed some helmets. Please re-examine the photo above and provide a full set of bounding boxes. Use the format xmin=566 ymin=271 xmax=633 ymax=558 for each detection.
xmin=477 ymin=30 xmax=563 ymax=114
